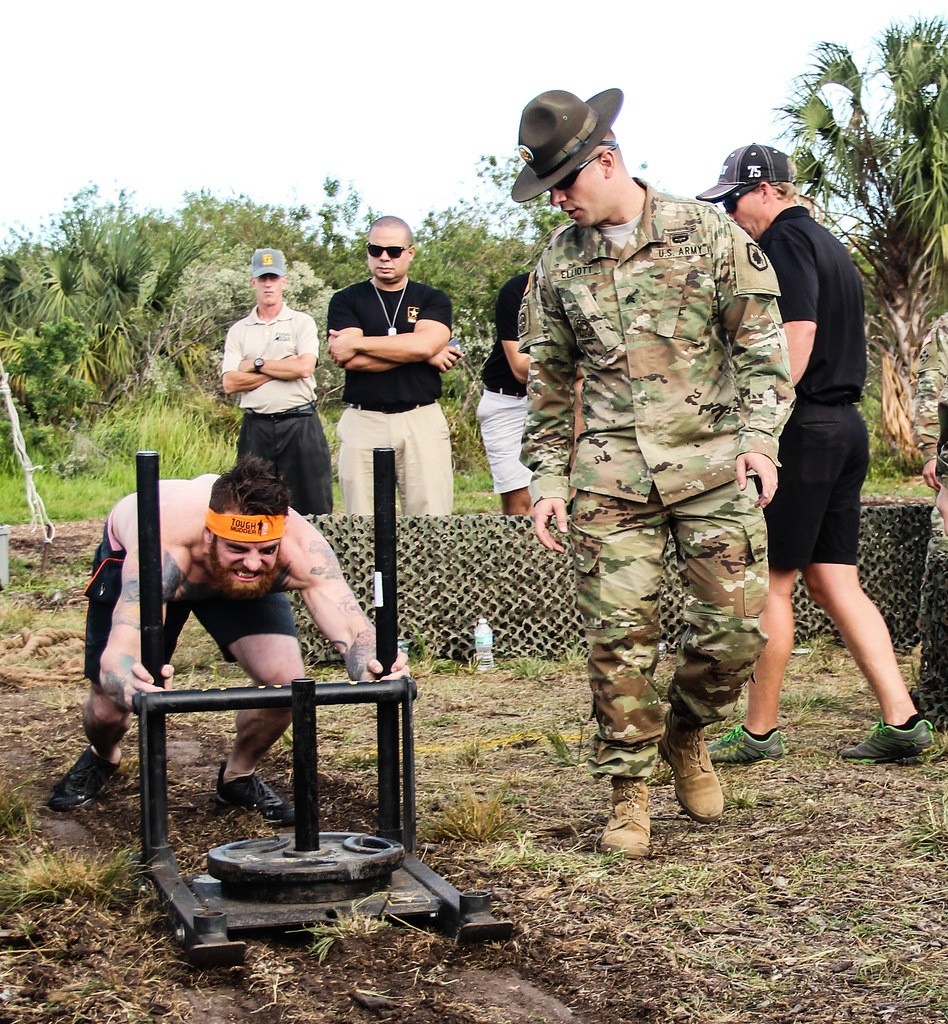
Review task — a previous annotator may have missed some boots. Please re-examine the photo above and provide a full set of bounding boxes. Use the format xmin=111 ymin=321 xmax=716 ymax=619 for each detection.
xmin=658 ymin=727 xmax=725 ymax=822
xmin=600 ymin=776 xmax=653 ymax=861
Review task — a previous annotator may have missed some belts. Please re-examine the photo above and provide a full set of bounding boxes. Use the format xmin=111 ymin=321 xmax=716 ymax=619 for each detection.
xmin=246 ymin=401 xmax=317 ymax=419
xmin=348 ymin=400 xmax=435 ymax=414
xmin=486 ymin=386 xmax=527 ymax=397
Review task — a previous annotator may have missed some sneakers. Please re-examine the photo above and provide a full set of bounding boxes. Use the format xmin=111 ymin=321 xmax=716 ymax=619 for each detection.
xmin=708 ymin=723 xmax=784 ymax=767
xmin=46 ymin=744 xmax=121 ymax=812
xmin=217 ymin=761 xmax=296 ymax=824
xmin=840 ymin=716 xmax=935 ymax=765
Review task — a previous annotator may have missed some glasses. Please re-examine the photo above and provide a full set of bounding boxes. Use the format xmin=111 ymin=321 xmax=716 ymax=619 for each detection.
xmin=722 ymin=181 xmax=781 ymax=214
xmin=366 ymin=242 xmax=413 ymax=258
xmin=554 ymin=145 xmax=618 ymax=190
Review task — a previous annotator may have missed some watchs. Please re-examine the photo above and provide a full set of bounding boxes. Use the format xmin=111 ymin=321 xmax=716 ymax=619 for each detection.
xmin=253 ymin=357 xmax=265 ymax=373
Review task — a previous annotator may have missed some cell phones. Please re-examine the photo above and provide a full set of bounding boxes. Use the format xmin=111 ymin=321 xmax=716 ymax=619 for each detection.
xmin=446 ymin=338 xmax=463 ymax=359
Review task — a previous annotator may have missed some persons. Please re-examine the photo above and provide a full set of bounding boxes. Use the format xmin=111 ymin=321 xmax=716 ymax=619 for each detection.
xmin=49 ymin=455 xmax=414 ymax=833
xmin=222 ymin=246 xmax=335 ymax=515
xmin=508 ymin=84 xmax=800 ymax=855
xmin=321 ymin=216 xmax=464 ymax=518
xmin=473 ymin=272 xmax=533 ymax=517
xmin=696 ymin=142 xmax=937 ymax=763
xmin=907 ymin=309 xmax=948 ymax=724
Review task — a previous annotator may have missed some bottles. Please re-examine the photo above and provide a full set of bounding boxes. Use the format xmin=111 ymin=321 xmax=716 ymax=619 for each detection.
xmin=474 ymin=618 xmax=494 ymax=671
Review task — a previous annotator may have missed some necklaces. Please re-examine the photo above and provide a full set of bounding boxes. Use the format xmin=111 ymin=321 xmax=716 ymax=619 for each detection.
xmin=372 ymin=279 xmax=407 ymax=336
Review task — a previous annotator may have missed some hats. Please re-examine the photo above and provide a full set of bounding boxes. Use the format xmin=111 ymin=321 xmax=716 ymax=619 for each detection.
xmin=511 ymin=88 xmax=624 ymax=203
xmin=695 ymin=143 xmax=798 ymax=203
xmin=251 ymin=249 xmax=286 ymax=277
